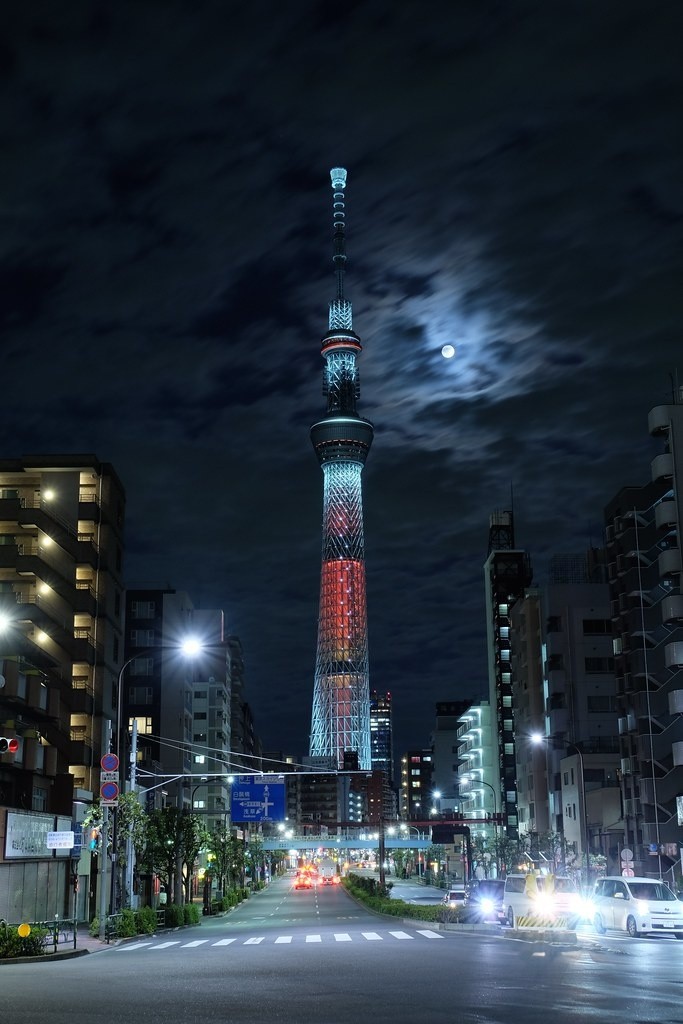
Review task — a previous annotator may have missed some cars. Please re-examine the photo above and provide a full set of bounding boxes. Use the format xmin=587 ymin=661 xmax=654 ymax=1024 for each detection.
xmin=441 ymin=879 xmax=506 ymax=919
xmin=295 ymin=859 xmax=340 ymax=887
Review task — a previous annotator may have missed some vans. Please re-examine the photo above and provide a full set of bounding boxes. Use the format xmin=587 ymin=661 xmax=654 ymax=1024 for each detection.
xmin=502 ymin=874 xmax=584 ymax=930
xmin=590 ymin=877 xmax=683 ymax=940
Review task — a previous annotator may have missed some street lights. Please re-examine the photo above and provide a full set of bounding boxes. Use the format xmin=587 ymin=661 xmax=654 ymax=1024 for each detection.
xmin=110 ymin=641 xmax=200 ymax=917
xmin=460 ymin=778 xmax=498 ymax=879
xmin=191 ymin=775 xmax=234 ymax=820
xmin=530 ymin=732 xmax=590 ymax=885
xmin=401 ymin=824 xmax=420 ymax=878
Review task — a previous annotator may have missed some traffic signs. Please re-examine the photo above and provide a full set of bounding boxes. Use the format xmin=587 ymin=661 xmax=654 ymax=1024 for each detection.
xmin=229 ymin=776 xmax=287 ymax=823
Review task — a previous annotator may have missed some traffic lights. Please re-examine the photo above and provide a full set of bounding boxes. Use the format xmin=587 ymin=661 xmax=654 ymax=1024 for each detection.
xmin=0 ymin=737 xmax=19 ymax=753
